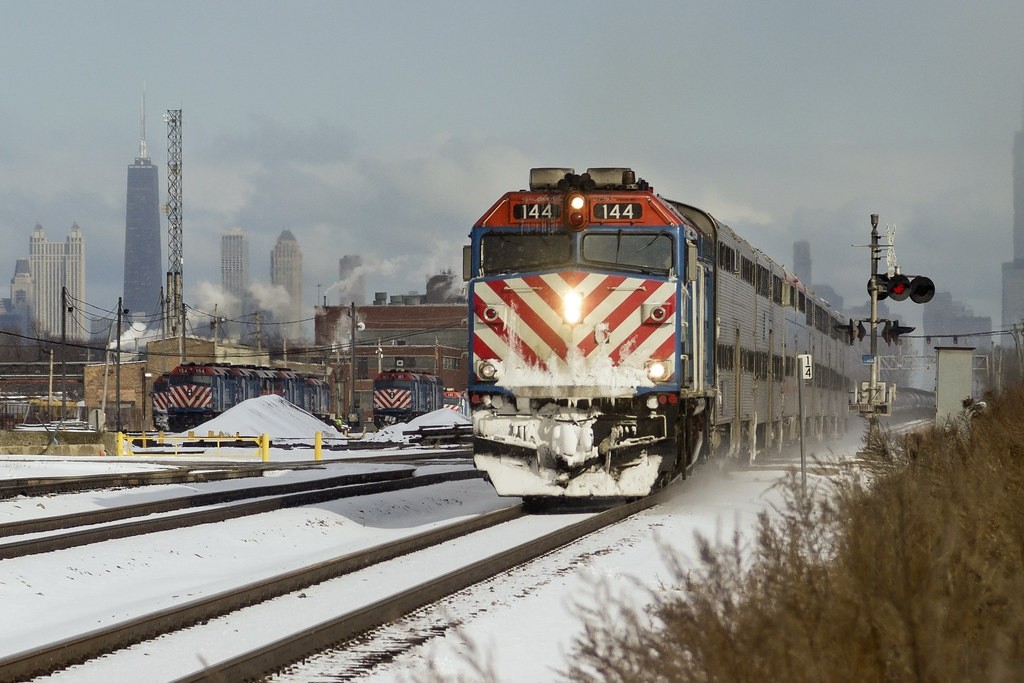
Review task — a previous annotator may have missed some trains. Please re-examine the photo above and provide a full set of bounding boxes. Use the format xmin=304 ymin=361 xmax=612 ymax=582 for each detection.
xmin=461 ymin=167 xmax=937 ymax=515
xmin=150 ymin=361 xmax=333 ymax=447
xmin=372 ymin=369 xmax=471 ymax=431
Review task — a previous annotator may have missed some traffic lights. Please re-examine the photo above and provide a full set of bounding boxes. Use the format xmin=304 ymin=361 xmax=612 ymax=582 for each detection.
xmin=909 ymin=275 xmax=935 ymax=304
xmin=833 ymin=318 xmax=857 ymax=345
xmin=886 ymin=275 xmax=912 ymax=301
xmin=892 ymin=319 xmax=916 ymax=344
xmin=883 ymin=320 xmax=906 ymax=346
